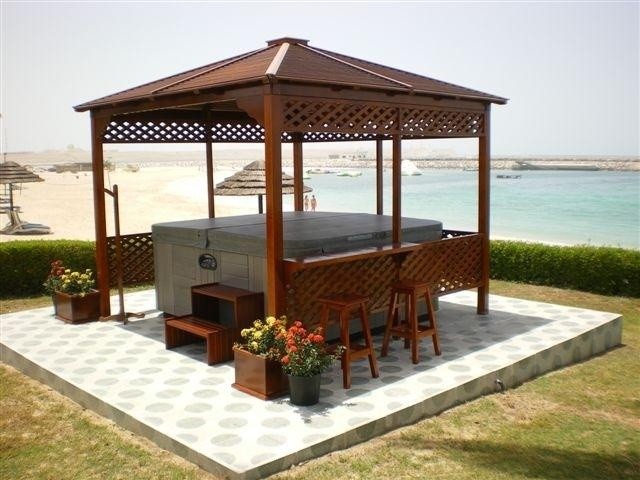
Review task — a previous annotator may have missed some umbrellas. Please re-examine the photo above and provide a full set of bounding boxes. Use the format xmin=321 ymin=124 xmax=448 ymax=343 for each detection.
xmin=0 ymin=161 xmax=44 ymax=224
xmin=214 ymin=158 xmax=313 ymax=215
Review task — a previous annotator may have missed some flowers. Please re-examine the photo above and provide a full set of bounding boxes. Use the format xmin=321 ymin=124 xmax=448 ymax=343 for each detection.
xmin=232 ymin=314 xmax=347 ymax=377
xmin=41 ymin=260 xmax=97 ymax=298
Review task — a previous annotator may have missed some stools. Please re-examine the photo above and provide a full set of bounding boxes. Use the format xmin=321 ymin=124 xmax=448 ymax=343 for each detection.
xmin=164 ymin=282 xmax=265 ymax=366
xmin=316 ymin=280 xmax=441 ymax=389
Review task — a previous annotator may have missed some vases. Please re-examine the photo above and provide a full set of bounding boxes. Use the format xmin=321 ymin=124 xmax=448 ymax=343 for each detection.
xmin=230 ymin=348 xmax=321 ymax=405
xmin=52 ymin=289 xmax=101 ymax=324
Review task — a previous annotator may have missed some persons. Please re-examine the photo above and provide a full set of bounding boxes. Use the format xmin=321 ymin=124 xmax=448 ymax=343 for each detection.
xmin=303 ymin=193 xmax=318 ymax=212
